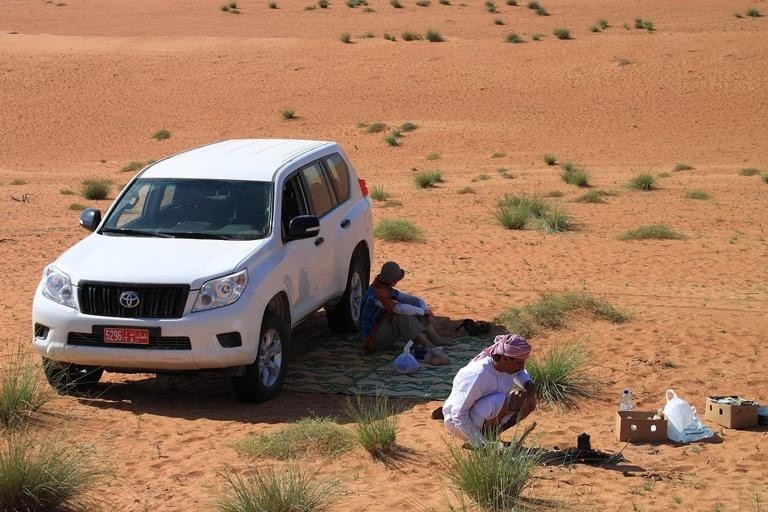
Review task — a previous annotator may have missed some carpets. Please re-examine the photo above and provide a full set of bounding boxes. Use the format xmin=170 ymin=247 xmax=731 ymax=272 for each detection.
xmin=281 ymin=333 xmax=499 ymax=399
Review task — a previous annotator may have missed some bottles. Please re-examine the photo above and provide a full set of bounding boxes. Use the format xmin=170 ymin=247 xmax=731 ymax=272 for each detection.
xmin=620 ymin=389 xmax=632 ymax=412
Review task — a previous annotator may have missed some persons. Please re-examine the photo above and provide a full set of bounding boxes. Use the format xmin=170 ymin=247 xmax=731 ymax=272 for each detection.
xmin=441 ymin=332 xmax=540 ymax=454
xmin=358 ymin=261 xmax=457 ymax=367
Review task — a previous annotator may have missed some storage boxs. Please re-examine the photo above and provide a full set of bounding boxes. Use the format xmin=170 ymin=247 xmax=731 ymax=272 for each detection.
xmin=705 ymin=394 xmax=757 ymax=429
xmin=616 ymin=410 xmax=667 ymax=444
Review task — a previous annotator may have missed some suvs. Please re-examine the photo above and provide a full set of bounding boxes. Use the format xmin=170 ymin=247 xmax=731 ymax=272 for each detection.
xmin=31 ymin=137 xmax=376 ymax=404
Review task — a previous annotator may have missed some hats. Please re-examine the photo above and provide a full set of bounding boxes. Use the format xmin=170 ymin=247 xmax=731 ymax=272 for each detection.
xmin=380 ymin=261 xmax=405 ymax=281
